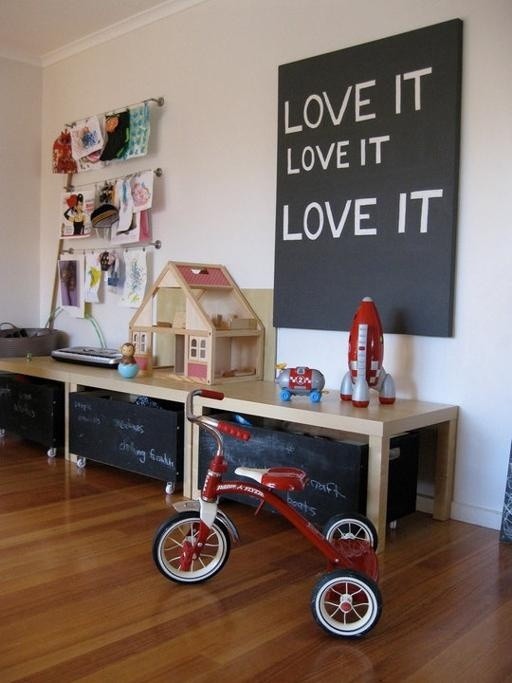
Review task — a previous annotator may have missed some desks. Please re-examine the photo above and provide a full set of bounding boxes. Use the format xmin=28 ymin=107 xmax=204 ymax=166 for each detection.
xmin=0 ymin=356 xmax=460 ymax=557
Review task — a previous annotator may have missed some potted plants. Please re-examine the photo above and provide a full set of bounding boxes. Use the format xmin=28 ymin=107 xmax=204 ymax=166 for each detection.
xmin=0 ymin=308 xmax=104 ymax=356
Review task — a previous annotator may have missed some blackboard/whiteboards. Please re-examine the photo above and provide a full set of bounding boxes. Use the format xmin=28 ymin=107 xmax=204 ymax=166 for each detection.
xmin=0 ymin=374 xmax=54 ymax=449
xmin=68 ymin=392 xmax=177 ymax=481
xmin=199 ymin=416 xmax=362 ymax=545
